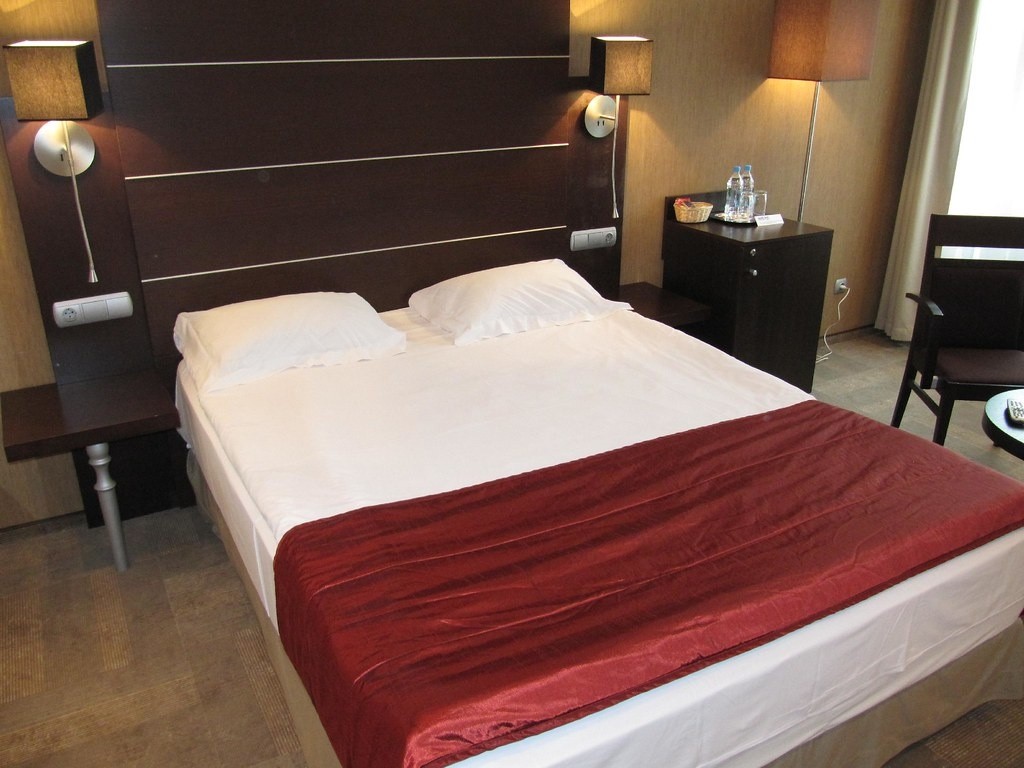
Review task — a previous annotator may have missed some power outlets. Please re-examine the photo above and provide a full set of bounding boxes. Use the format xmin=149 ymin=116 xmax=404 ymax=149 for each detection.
xmin=834 ymin=278 xmax=847 ymax=293
xmin=53 ymin=292 xmax=136 ymax=329
xmin=570 ymin=227 xmax=617 ymax=251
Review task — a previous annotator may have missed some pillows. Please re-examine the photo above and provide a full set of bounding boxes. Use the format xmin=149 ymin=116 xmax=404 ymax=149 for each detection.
xmin=173 ymin=291 xmax=408 ymax=390
xmin=408 ymin=258 xmax=635 ymax=349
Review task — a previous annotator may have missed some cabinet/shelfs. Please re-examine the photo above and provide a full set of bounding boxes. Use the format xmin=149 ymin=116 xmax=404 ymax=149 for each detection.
xmin=664 ymin=186 xmax=834 ymax=394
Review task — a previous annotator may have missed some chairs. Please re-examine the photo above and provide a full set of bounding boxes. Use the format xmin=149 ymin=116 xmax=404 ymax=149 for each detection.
xmin=890 ymin=214 xmax=1024 ymax=447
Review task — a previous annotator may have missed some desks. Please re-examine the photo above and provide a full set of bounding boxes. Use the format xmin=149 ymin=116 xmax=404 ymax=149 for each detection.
xmin=982 ymin=389 xmax=1024 ymax=462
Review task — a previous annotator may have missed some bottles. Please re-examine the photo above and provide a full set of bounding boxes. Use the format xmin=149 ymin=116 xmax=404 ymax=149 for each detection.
xmin=740 ymin=165 xmax=754 ymax=194
xmin=724 ymin=166 xmax=743 ymax=220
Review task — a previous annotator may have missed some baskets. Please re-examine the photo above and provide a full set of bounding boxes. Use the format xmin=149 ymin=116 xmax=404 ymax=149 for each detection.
xmin=674 ymin=201 xmax=713 ymax=223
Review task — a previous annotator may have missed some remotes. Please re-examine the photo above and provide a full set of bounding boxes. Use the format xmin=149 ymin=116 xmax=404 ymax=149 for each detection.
xmin=1007 ymin=399 xmax=1024 ymax=424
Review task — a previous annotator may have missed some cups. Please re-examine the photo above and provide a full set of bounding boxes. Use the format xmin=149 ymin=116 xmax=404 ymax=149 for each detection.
xmin=754 ymin=190 xmax=768 ymax=216
xmin=739 ymin=192 xmax=755 ymax=218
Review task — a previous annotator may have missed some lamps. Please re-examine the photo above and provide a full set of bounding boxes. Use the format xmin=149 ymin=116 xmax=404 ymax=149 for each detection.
xmin=768 ymin=0 xmax=882 ymax=224
xmin=582 ymin=34 xmax=654 ymax=219
xmin=1 ymin=38 xmax=110 ymax=287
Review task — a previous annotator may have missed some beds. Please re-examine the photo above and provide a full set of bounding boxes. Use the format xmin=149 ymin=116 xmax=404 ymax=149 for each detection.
xmin=0 ymin=0 xmax=1024 ymax=768
